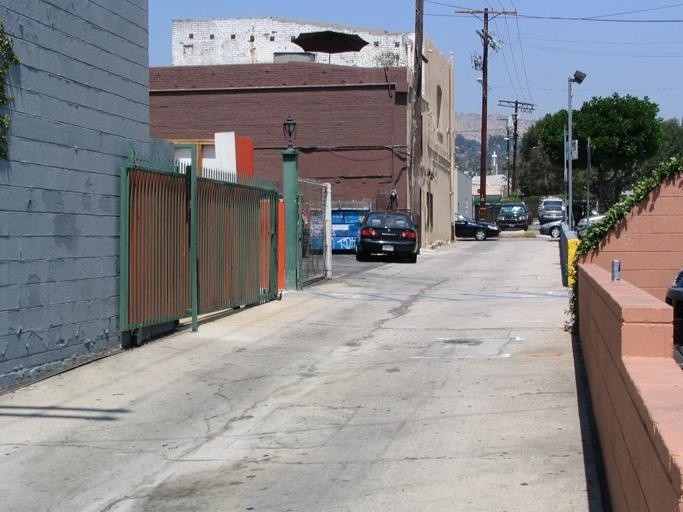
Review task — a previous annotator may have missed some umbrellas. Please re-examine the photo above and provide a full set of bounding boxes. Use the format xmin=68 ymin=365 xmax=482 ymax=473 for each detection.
xmin=291 ymin=29 xmax=368 ymax=64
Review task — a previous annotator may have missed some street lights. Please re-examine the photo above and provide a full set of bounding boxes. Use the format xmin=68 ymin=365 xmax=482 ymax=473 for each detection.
xmin=477 ymin=77 xmax=491 ymax=91
xmin=568 ymin=70 xmax=587 ymax=231
xmin=281 ymin=114 xmax=302 ymax=291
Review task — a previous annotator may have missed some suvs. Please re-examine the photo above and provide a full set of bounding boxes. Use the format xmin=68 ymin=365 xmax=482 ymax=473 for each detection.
xmin=495 ymin=202 xmax=529 ymax=231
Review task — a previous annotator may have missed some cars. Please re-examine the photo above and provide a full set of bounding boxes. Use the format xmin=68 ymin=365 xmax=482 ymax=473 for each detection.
xmin=539 ymin=210 xmax=567 ymax=238
xmin=577 ymin=211 xmax=608 ymax=230
xmin=537 ymin=195 xmax=565 ymax=225
xmin=664 ymin=266 xmax=683 ymax=346
xmin=524 ymin=204 xmax=534 ymax=225
xmin=455 ymin=213 xmax=501 ymax=241
xmin=355 ymin=211 xmax=419 ymax=263
